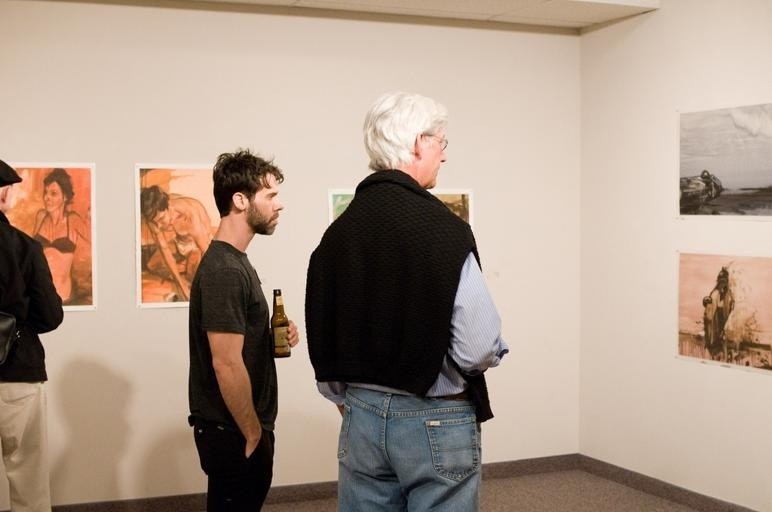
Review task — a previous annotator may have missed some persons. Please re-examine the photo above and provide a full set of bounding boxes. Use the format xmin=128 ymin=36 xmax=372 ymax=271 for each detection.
xmin=303 ymin=93 xmax=513 ymax=511
xmin=704 ymin=268 xmax=736 ymax=356
xmin=185 ymin=144 xmax=303 ymax=511
xmin=141 ymin=184 xmax=214 ymax=301
xmin=1 ymin=157 xmax=65 ymax=511
xmin=699 ymin=170 xmax=720 ymax=208
xmin=29 ymin=169 xmax=92 ymax=311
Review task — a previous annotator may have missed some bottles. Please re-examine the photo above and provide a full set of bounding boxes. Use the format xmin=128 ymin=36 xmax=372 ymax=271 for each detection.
xmin=267 ymin=286 xmax=292 ymax=360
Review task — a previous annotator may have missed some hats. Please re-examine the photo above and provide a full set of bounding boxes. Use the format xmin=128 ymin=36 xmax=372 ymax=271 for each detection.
xmin=0 ymin=160 xmax=23 ymax=187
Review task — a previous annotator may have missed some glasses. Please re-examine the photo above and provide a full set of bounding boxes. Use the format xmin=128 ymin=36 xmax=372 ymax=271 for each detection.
xmin=426 ymin=133 xmax=449 ymax=152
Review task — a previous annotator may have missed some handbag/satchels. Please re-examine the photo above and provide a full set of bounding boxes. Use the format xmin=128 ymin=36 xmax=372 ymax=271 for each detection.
xmin=0 ymin=313 xmax=19 ymax=365
